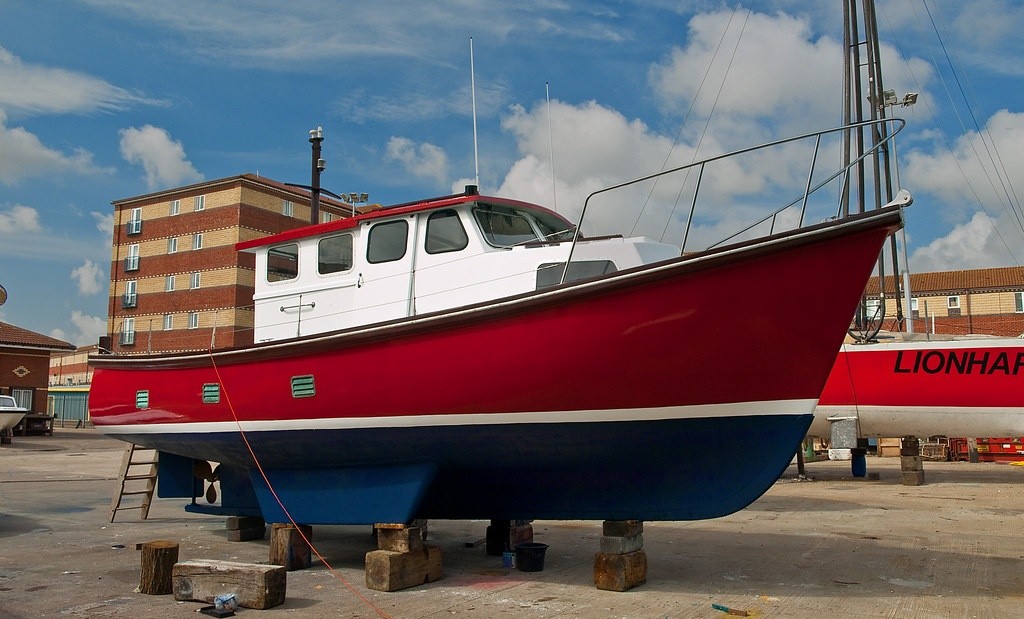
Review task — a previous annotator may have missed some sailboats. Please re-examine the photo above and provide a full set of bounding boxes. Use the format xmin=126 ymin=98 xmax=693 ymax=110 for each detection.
xmin=807 ymin=7 xmax=1024 ymax=450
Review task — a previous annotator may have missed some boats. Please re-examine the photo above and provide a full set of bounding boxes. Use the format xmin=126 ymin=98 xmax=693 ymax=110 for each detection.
xmin=87 ymin=119 xmax=913 ymax=524
xmin=0 ymin=395 xmax=27 ymax=432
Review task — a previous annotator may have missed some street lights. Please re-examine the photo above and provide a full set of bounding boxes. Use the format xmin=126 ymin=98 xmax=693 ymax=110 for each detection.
xmin=340 ymin=193 xmax=368 ymax=217
xmin=867 ymin=88 xmax=918 ymax=332
xmin=309 ymin=127 xmax=326 ymax=225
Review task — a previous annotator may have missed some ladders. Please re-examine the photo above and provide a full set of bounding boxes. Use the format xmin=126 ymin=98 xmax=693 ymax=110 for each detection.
xmin=109 ymin=443 xmax=159 ymax=523
